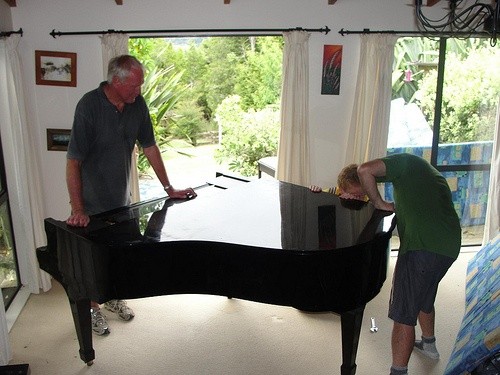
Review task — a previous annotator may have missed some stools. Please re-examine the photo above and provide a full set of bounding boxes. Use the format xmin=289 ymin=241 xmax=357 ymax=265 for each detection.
xmin=258 ymin=156 xmax=277 ymax=179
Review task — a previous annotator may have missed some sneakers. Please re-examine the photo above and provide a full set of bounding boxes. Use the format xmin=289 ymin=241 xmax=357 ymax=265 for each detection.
xmin=105 ymin=298 xmax=135 ymax=322
xmin=90 ymin=308 xmax=111 ymax=335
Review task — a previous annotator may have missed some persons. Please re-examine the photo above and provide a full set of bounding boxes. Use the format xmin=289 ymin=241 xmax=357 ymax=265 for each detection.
xmin=309 ymin=163 xmax=369 ymax=202
xmin=357 ymin=153 xmax=461 ymax=375
xmin=66 ymin=55 xmax=197 ymax=334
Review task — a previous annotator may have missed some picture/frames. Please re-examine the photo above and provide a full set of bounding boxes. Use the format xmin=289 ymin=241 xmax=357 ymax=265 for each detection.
xmin=33 ymin=49 xmax=78 ymax=88
xmin=46 ymin=128 xmax=72 ymax=152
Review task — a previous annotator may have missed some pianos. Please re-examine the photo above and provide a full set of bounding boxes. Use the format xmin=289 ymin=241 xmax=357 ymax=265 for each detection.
xmin=34 ymin=168 xmax=394 ymax=374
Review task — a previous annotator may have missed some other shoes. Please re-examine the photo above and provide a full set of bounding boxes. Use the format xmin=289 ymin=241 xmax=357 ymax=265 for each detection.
xmin=414 ymin=336 xmax=439 ymax=360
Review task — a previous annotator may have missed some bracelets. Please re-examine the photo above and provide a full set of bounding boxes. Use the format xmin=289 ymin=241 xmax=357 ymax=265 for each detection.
xmin=164 ymin=184 xmax=172 ymax=191
